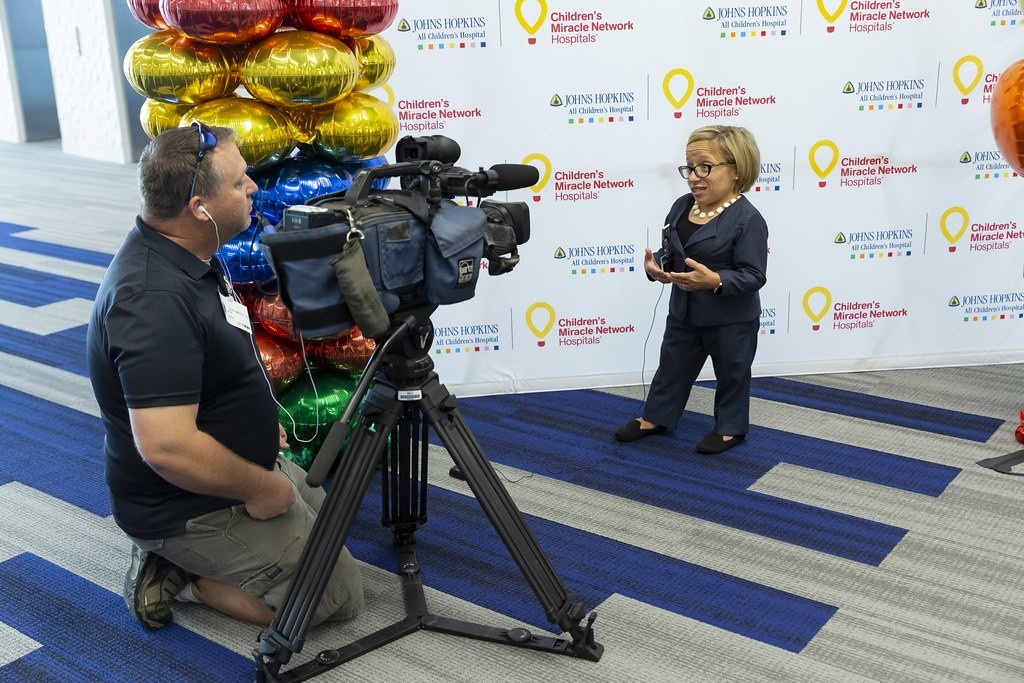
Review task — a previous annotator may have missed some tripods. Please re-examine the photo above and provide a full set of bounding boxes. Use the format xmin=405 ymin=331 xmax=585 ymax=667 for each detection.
xmin=252 ymin=314 xmax=604 ymax=683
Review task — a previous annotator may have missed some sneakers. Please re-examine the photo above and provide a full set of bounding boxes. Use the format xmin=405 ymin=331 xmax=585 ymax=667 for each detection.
xmin=123 ymin=542 xmax=200 ymax=630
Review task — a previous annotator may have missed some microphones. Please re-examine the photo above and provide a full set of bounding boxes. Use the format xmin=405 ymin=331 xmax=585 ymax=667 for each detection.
xmin=474 ymin=163 xmax=539 ymax=198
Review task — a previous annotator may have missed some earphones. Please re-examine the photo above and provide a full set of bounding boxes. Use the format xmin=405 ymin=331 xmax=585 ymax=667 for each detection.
xmin=197 ymin=206 xmax=213 ymax=220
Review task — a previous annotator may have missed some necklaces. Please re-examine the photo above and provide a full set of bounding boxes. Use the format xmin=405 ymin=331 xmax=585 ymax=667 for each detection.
xmin=692 ymin=196 xmax=742 ymax=218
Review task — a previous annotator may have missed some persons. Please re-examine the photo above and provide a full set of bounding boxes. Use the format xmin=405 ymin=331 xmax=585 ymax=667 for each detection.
xmin=613 ymin=126 xmax=768 ymax=454
xmin=86 ymin=126 xmax=363 ymax=632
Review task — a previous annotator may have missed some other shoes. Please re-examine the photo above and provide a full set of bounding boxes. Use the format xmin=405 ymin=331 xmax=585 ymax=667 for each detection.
xmin=613 ymin=418 xmax=668 ymax=442
xmin=697 ymin=430 xmax=746 ymax=453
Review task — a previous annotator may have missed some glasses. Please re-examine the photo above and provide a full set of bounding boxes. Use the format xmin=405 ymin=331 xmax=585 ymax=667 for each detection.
xmin=185 ymin=120 xmax=217 ymax=205
xmin=678 ymin=161 xmax=736 ymax=180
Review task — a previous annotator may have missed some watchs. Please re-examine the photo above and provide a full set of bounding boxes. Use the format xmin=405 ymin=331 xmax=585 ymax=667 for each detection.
xmin=713 ymin=281 xmax=723 ymax=293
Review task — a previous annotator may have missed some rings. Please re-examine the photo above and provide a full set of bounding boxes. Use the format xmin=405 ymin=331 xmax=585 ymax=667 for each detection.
xmin=683 ymin=284 xmax=685 ymax=290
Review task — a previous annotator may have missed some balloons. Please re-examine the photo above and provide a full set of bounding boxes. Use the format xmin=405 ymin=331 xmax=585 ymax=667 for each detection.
xmin=124 ymin=0 xmax=397 ymax=474
xmin=990 ymin=60 xmax=1024 ymax=178
